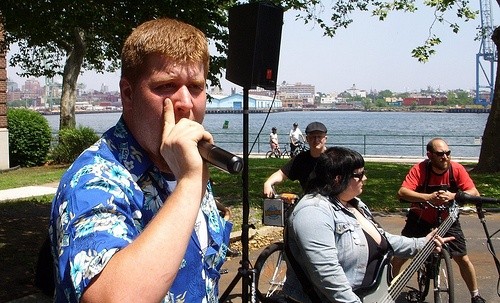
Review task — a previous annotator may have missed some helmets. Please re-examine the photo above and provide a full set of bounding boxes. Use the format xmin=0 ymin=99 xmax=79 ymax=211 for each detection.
xmin=293 ymin=122 xmax=298 ymax=126
xmin=271 ymin=126 xmax=277 ymax=130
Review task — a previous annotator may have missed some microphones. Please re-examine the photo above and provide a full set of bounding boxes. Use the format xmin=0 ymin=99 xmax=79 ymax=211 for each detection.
xmin=197 ymin=140 xmax=244 ymax=175
xmin=455 ymin=191 xmax=500 ymax=204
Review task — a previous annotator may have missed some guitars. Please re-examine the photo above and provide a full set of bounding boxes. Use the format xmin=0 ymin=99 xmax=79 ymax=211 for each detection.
xmin=354 ymin=199 xmax=464 ymax=303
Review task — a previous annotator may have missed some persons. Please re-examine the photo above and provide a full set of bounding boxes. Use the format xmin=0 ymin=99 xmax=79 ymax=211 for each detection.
xmin=390 ymin=138 xmax=489 ymax=303
xmin=264 ymin=122 xmax=328 ymax=198
xmin=269 ymin=127 xmax=281 ymax=158
xmin=289 ymin=122 xmax=305 ymax=157
xmin=283 ymin=148 xmax=455 ymax=303
xmin=50 ymin=20 xmax=233 ymax=303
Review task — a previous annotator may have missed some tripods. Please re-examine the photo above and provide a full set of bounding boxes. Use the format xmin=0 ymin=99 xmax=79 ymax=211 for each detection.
xmin=218 ymin=87 xmax=268 ymax=303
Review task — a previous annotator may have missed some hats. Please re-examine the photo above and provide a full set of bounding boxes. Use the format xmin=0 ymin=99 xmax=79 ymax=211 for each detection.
xmin=305 ymin=122 xmax=327 ymax=135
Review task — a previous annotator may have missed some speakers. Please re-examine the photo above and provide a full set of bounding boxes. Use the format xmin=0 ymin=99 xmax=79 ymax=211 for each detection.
xmin=226 ymin=2 xmax=283 ymax=90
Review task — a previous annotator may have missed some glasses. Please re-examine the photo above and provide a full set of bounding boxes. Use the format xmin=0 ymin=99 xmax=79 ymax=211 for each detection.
xmin=352 ymin=170 xmax=367 ymax=178
xmin=432 ymin=150 xmax=451 ymax=156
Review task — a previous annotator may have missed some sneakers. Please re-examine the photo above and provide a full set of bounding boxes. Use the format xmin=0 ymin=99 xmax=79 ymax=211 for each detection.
xmin=470 ymin=294 xmax=486 ymax=303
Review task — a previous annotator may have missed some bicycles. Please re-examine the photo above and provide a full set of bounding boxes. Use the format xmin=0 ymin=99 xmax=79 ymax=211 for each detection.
xmin=381 ymin=194 xmax=461 ymax=303
xmin=265 ymin=140 xmax=311 ymax=159
xmin=251 ymin=187 xmax=330 ymax=302
